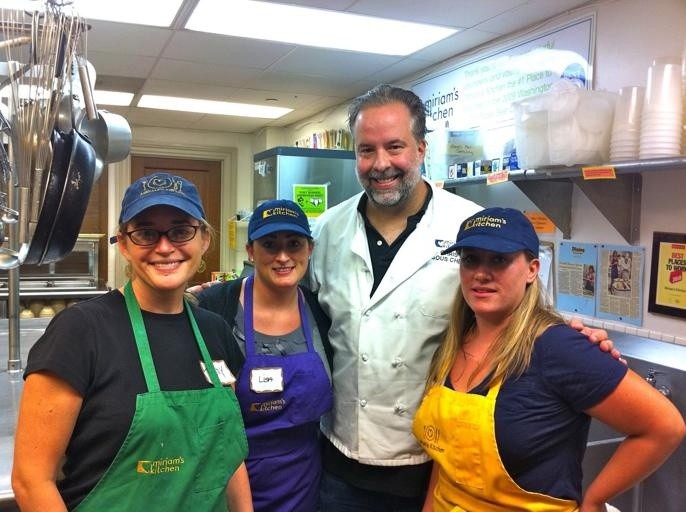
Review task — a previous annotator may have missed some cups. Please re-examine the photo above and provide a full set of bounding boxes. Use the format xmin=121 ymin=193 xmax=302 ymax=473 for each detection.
xmin=609 ymin=63 xmax=683 ymax=163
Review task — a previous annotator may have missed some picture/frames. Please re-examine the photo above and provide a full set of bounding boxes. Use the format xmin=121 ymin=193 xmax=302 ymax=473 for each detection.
xmin=648 ymin=231 xmax=686 ymax=318
xmin=410 ymin=10 xmax=597 ymax=181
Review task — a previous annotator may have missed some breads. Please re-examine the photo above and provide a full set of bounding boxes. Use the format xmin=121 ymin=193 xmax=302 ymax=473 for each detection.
xmin=19 ymin=299 xmax=80 ymax=318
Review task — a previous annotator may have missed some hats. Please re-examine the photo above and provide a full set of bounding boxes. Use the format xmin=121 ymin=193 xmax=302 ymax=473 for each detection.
xmin=441 ymin=207 xmax=538 ymax=257
xmin=248 ymin=200 xmax=313 ymax=240
xmin=119 ymin=173 xmax=206 ymax=227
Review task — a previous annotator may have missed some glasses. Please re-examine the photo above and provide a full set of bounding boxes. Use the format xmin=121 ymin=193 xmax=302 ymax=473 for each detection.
xmin=122 ymin=225 xmax=201 ymax=246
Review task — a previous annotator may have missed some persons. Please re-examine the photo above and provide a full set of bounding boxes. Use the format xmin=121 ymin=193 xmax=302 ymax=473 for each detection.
xmin=411 ymin=207 xmax=686 ymax=512
xmin=193 ymin=199 xmax=334 ymax=512
xmin=190 ymin=85 xmax=628 ymax=512
xmin=11 ymin=173 xmax=254 ymax=512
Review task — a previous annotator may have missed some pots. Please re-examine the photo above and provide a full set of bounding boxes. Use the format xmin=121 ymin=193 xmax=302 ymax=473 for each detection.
xmin=80 ymin=109 xmax=133 ymax=164
xmin=19 ymin=27 xmax=96 ymax=267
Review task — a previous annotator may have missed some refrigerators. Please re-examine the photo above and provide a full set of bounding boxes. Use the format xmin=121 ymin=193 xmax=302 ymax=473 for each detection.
xmin=253 ymin=146 xmax=364 ymax=228
